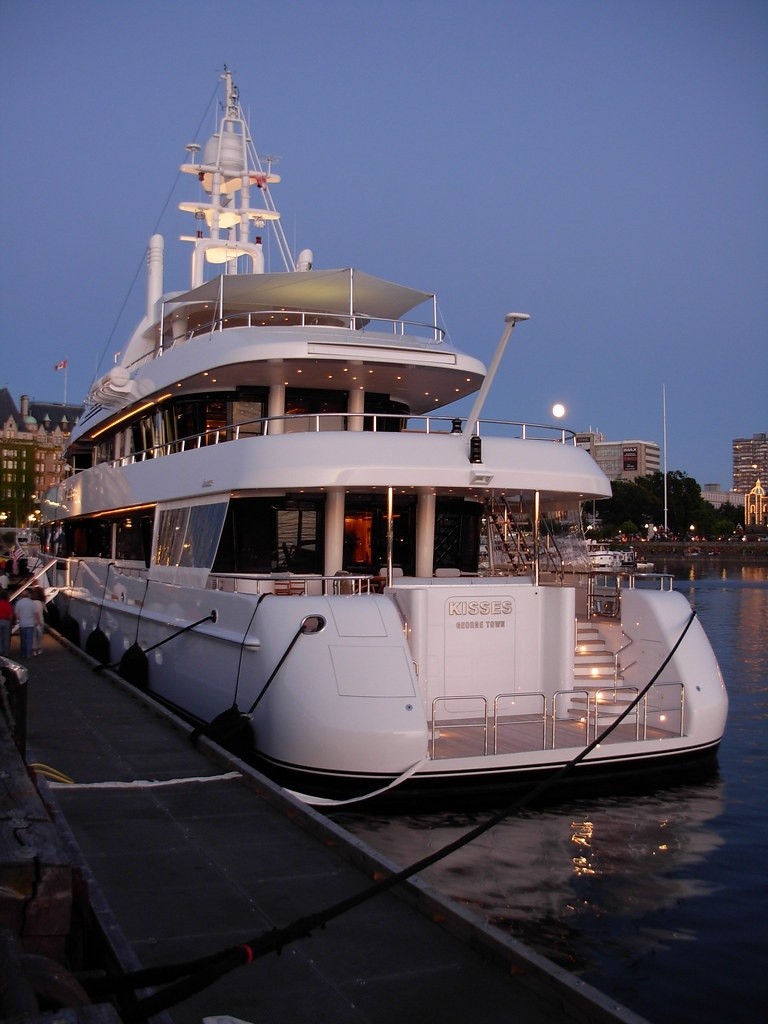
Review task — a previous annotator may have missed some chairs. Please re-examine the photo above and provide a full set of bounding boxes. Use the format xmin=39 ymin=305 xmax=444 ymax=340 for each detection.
xmin=237 ymin=568 xmax=461 ymax=595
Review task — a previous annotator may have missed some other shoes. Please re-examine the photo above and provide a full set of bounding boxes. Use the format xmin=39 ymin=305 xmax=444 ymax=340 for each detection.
xmin=22 ymin=656 xmax=32 ymax=659
xmin=32 ymin=651 xmax=42 ymax=656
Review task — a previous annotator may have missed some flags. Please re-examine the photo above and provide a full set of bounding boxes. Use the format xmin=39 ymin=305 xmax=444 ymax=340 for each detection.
xmin=55 ymin=359 xmax=67 ymax=371
xmin=11 ymin=540 xmax=24 ymax=572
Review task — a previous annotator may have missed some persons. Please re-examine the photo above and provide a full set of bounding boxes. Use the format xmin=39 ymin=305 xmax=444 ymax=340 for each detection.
xmin=0 ymin=572 xmax=45 ymax=660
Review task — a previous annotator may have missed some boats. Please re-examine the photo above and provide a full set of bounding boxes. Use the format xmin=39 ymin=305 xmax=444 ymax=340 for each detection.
xmin=480 ymin=536 xmax=654 ymax=571
xmin=13 ymin=64 xmax=734 ymax=789
